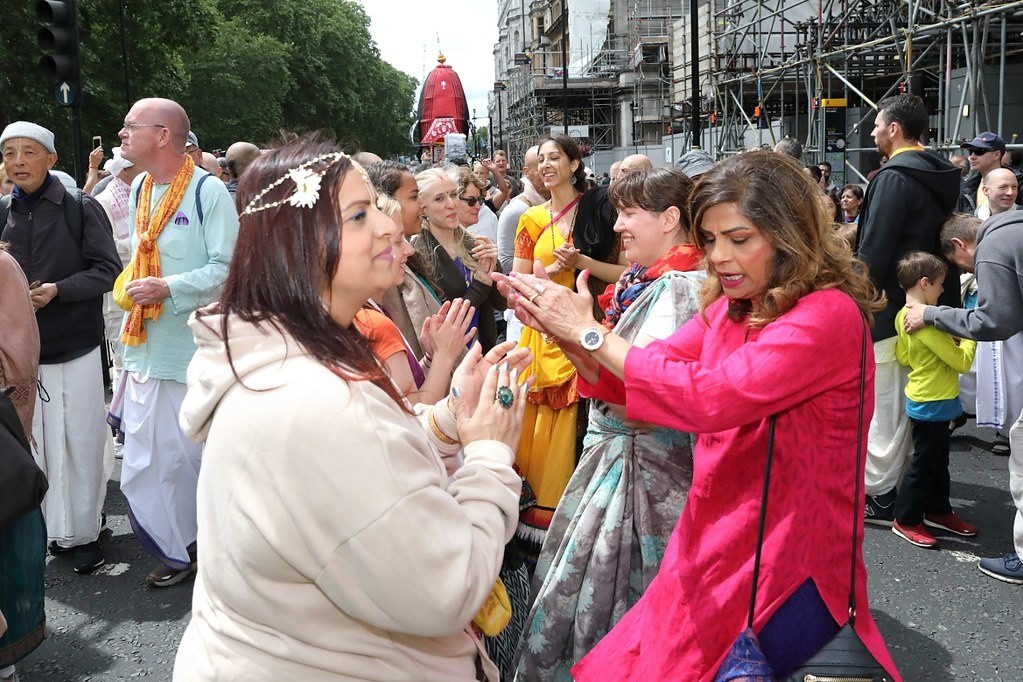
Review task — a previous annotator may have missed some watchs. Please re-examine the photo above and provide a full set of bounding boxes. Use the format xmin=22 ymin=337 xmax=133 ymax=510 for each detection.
xmin=578 ymin=325 xmax=611 ymax=358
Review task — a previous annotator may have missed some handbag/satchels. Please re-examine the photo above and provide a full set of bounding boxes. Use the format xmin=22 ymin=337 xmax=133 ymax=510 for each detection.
xmin=711 ymin=621 xmax=895 ymax=682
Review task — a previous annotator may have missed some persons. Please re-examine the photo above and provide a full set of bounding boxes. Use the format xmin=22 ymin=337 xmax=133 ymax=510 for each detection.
xmin=0 ymin=97 xmax=1023 ymax=682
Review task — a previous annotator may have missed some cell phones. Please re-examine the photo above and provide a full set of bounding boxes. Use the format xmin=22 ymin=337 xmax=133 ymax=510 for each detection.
xmin=93 ymin=136 xmax=101 ymax=150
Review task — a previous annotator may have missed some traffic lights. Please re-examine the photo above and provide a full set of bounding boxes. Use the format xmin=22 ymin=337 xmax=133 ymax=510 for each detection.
xmin=34 ymin=0 xmax=80 ymax=82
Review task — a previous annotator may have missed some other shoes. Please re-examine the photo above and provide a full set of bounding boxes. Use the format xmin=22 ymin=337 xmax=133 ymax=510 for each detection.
xmin=0 ymin=672 xmax=19 ymax=682
xmin=948 ymin=411 xmax=967 ymax=437
xmin=990 ymin=434 xmax=1011 ymax=454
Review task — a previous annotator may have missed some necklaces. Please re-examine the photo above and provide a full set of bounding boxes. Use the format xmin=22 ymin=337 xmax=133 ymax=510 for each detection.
xmin=550 ymin=198 xmax=578 ymax=251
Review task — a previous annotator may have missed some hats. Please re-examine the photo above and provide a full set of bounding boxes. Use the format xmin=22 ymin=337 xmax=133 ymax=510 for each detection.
xmin=185 ymin=130 xmax=200 ymax=148
xmin=674 ymin=149 xmax=716 ymax=181
xmin=0 ymin=120 xmax=57 ymax=155
xmin=960 ymin=132 xmax=1006 ymax=154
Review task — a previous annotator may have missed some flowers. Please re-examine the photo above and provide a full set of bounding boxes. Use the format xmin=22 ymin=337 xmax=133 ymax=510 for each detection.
xmin=283 ymin=164 xmax=323 ymax=209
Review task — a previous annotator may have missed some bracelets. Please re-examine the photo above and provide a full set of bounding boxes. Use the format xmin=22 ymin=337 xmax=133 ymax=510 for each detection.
xmin=428 ymin=406 xmax=461 ymax=446
xmin=88 ymin=166 xmax=99 ymax=170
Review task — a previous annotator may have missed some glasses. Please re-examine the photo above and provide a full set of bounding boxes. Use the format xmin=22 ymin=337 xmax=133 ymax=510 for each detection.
xmin=969 ymin=147 xmax=996 ymax=157
xmin=122 ymin=121 xmax=165 ymax=131
xmin=459 ymin=196 xmax=485 ymax=206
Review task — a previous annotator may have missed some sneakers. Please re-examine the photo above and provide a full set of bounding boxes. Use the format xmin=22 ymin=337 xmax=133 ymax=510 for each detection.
xmin=923 ymin=508 xmax=977 ymax=536
xmin=47 ymin=510 xmax=109 ymax=554
xmin=72 ymin=535 xmax=104 ymax=572
xmin=890 ymin=518 xmax=937 ymax=549
xmin=146 ymin=545 xmax=197 ymax=587
xmin=862 ymin=492 xmax=897 ymax=527
xmin=978 ymin=554 xmax=1023 ymax=584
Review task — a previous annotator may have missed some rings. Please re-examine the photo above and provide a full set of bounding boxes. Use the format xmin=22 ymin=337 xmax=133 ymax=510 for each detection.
xmin=529 ymin=293 xmax=539 ymax=302
xmin=496 ymin=385 xmax=514 ymax=409
xmin=536 ymin=285 xmax=545 ymax=294
